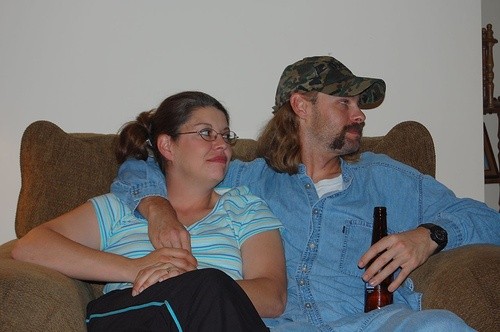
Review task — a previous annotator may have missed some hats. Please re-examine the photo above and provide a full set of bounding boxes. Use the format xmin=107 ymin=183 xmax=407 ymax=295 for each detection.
xmin=272 ymin=56 xmax=386 ymax=115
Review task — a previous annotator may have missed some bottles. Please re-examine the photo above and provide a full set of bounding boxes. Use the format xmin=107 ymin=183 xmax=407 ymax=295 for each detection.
xmin=365 ymin=207 xmax=394 ymax=312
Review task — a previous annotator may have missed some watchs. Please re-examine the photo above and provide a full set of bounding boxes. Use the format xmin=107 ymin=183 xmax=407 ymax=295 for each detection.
xmin=417 ymin=223 xmax=448 ymax=255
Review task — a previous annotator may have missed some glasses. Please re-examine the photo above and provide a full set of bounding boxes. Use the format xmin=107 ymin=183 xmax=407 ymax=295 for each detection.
xmin=175 ymin=128 xmax=239 ymax=144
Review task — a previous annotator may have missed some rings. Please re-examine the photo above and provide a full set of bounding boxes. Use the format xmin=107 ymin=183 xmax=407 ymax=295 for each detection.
xmin=166 ymin=267 xmax=173 ymax=272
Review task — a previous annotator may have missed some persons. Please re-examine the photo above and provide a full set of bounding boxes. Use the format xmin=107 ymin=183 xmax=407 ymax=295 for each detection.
xmin=110 ymin=55 xmax=500 ymax=332
xmin=12 ymin=91 xmax=288 ymax=332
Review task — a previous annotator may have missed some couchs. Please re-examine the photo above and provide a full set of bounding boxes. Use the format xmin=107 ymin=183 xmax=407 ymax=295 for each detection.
xmin=0 ymin=120 xmax=500 ymax=332
